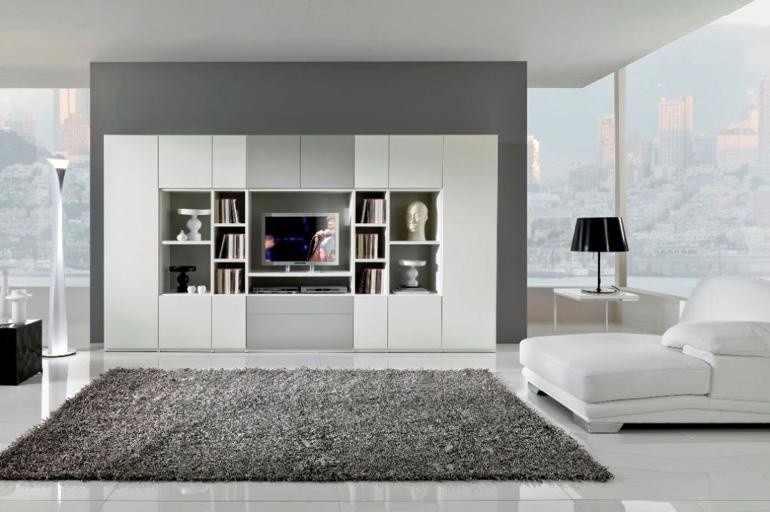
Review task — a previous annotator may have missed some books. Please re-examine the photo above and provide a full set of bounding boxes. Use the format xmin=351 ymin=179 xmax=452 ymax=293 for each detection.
xmin=356 ymin=196 xmax=386 ymax=294
xmin=215 ymin=198 xmax=244 ymax=295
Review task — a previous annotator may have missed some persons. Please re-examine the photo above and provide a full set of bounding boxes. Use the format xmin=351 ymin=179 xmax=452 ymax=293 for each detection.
xmin=264 ymin=235 xmax=275 ymax=263
xmin=405 ymin=200 xmax=428 ymax=241
xmin=311 ymin=217 xmax=336 ymax=262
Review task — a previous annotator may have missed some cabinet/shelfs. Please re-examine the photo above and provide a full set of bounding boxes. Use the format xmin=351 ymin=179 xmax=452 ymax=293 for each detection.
xmin=102 ymin=133 xmax=498 ymax=352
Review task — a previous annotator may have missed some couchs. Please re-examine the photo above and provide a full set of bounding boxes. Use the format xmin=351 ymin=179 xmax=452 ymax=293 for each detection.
xmin=517 ymin=274 xmax=770 ymax=434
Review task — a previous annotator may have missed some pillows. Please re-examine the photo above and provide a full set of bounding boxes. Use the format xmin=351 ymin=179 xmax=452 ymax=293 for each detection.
xmin=659 ymin=317 xmax=769 ymax=355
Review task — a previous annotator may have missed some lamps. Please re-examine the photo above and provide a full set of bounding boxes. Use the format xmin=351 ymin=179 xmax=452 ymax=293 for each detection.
xmin=569 ymin=214 xmax=629 ymax=294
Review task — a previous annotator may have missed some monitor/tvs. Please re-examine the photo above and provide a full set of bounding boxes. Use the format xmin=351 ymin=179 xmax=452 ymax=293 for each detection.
xmin=261 ymin=213 xmax=339 ymax=273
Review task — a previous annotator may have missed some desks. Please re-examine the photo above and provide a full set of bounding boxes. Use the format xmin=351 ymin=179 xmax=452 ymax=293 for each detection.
xmin=552 ymin=287 xmax=640 ymax=333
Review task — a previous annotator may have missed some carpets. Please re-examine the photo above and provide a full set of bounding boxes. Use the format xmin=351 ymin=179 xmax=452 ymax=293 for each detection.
xmin=1 ymin=364 xmax=613 ymax=481
